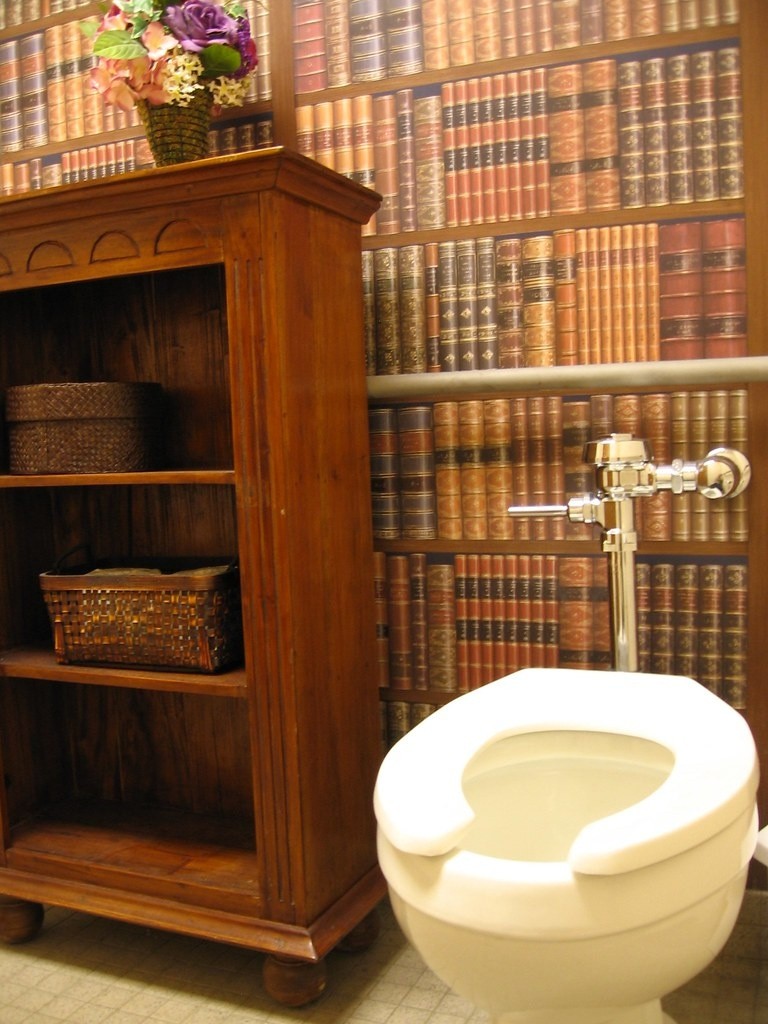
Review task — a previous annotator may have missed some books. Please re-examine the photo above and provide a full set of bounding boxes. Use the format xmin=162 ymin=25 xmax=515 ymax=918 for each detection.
xmin=295 ymin=46 xmax=749 ymax=238
xmin=370 ymin=552 xmax=749 ymax=711
xmin=295 ymin=0 xmax=739 ymax=96
xmin=369 ymin=387 xmax=748 ymax=543
xmin=361 ymin=218 xmax=746 ymax=378
xmin=377 ymin=702 xmax=434 ymax=750
xmin=0 ymin=0 xmax=274 ymax=199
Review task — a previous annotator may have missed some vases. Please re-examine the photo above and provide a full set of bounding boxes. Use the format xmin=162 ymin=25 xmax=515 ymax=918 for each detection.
xmin=134 ymin=84 xmax=211 ymax=167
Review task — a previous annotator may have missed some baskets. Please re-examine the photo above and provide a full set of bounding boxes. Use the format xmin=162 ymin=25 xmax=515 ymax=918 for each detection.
xmin=38 ymin=555 xmax=243 ymax=674
xmin=4 ymin=381 xmax=164 ymax=474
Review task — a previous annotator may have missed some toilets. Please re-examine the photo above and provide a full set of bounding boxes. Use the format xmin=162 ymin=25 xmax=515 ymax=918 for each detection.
xmin=371 ymin=666 xmax=761 ymax=1023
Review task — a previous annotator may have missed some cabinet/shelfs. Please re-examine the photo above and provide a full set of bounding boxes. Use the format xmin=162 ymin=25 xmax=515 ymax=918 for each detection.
xmin=0 ymin=149 xmax=384 ymax=1004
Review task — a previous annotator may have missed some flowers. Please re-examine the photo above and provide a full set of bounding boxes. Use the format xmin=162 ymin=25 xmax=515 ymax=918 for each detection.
xmin=79 ymin=0 xmax=259 ymax=111
